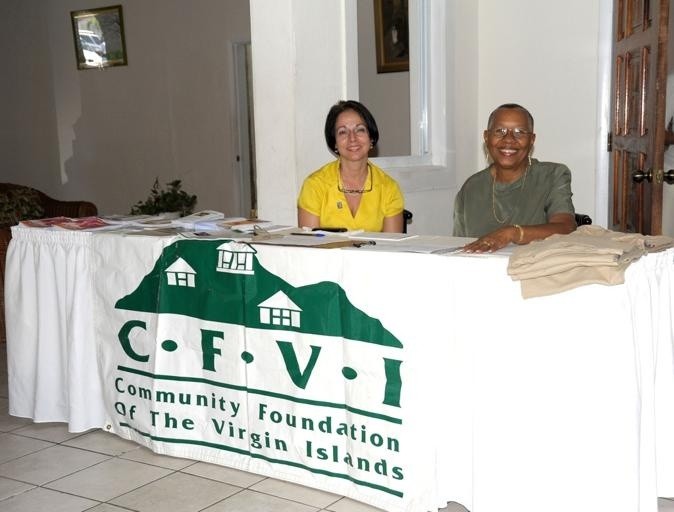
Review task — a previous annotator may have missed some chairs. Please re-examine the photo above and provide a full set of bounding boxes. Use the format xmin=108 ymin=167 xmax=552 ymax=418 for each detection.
xmin=0 ymin=183 xmax=99 ymax=280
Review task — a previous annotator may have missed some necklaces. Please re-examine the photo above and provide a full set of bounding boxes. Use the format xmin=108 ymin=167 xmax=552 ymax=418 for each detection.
xmin=492 ymin=163 xmax=529 ymax=224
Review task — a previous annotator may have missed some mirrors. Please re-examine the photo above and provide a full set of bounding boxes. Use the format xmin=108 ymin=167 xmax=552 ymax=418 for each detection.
xmin=343 ymin=0 xmax=425 ymax=173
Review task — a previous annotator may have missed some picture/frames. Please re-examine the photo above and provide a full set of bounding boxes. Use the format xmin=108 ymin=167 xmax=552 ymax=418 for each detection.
xmin=374 ymin=0 xmax=409 ymax=74
xmin=70 ymin=5 xmax=128 ymax=70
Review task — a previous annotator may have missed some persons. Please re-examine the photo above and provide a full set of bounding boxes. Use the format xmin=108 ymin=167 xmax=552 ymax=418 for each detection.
xmin=451 ymin=104 xmax=577 ymax=255
xmin=297 ymin=99 xmax=405 ymax=234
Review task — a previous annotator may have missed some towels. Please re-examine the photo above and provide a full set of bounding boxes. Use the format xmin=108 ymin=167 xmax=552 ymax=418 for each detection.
xmin=507 ymin=224 xmax=673 ymax=299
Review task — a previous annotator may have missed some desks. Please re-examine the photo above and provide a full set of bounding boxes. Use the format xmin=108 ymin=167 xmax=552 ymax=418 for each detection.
xmin=4 ymin=213 xmax=674 ymax=512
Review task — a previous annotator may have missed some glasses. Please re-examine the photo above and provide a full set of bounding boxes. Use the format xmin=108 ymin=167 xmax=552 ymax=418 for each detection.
xmin=336 ymin=163 xmax=373 ymax=194
xmin=491 ymin=126 xmax=533 ymax=138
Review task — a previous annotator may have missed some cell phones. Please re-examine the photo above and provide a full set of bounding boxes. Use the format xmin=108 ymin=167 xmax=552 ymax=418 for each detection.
xmin=312 ymin=228 xmax=347 ymax=232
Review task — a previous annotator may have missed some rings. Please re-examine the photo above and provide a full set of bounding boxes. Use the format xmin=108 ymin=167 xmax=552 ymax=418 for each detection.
xmin=484 ymin=241 xmax=492 ymax=250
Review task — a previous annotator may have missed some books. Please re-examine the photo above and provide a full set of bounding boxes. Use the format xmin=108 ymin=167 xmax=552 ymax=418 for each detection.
xmin=17 ymin=209 xmax=294 ymax=235
xmin=348 ymin=232 xmax=420 ymax=242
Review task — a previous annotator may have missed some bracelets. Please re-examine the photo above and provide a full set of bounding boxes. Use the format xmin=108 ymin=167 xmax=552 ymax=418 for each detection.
xmin=511 ymin=224 xmax=525 ymax=244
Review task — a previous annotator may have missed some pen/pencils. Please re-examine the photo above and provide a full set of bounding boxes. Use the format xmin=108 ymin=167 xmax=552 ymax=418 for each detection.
xmin=290 ymin=233 xmax=324 ymax=237
